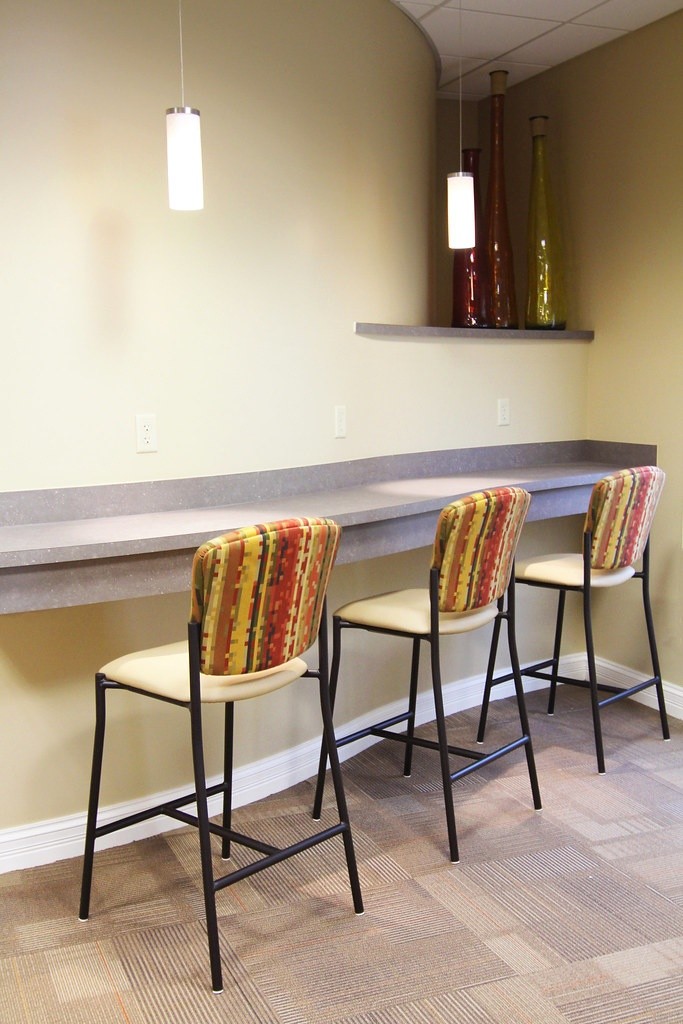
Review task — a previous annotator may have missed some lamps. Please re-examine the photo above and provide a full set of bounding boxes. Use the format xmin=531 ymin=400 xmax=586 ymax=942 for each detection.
xmin=447 ymin=0 xmax=476 ymax=249
xmin=166 ymin=1 xmax=204 ymax=210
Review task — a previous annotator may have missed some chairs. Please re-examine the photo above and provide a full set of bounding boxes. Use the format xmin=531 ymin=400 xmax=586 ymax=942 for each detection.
xmin=79 ymin=519 xmax=362 ymax=993
xmin=476 ymin=467 xmax=670 ymax=774
xmin=313 ymin=487 xmax=541 ymax=864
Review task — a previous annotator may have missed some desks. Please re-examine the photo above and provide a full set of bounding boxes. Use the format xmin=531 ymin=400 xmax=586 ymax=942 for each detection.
xmin=0 ymin=462 xmax=629 ymax=615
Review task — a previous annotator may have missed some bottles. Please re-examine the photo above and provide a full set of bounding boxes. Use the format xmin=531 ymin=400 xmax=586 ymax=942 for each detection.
xmin=480 ymin=70 xmax=519 ymax=328
xmin=523 ymin=115 xmax=567 ymax=329
xmin=451 ymin=148 xmax=480 ymax=328
